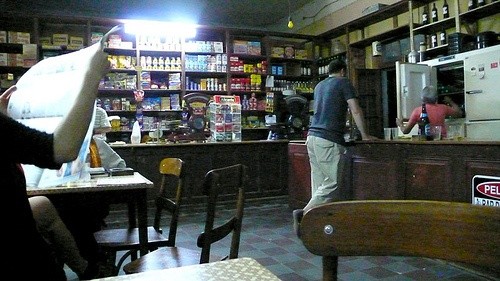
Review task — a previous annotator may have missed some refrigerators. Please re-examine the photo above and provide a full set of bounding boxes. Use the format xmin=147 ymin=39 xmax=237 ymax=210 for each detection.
xmin=394 ymin=44 xmax=500 ymax=139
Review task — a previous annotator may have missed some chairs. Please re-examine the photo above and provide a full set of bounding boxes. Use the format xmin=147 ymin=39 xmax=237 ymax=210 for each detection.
xmin=123 ymin=164 xmax=250 ymax=273
xmin=94 ymin=158 xmax=185 ymax=274
xmin=299 ymin=201 xmax=500 ymax=281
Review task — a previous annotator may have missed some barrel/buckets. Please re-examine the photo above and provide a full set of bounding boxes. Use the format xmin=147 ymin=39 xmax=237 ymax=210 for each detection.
xmin=473 ymin=31 xmax=498 ymax=50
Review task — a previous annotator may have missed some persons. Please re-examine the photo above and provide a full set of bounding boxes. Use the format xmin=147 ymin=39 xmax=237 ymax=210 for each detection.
xmin=93 ymin=107 xmax=112 ymax=142
xmin=293 ymin=60 xmax=379 ymax=234
xmin=50 ymin=137 xmax=127 ymax=260
xmin=396 ymin=85 xmax=458 ymax=138
xmin=0 ymin=44 xmax=111 ymax=281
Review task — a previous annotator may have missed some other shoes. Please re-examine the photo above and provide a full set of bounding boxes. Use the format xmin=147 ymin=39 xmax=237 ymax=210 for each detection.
xmin=293 ymin=208 xmax=303 ymax=239
xmin=81 ymin=261 xmax=118 ymax=280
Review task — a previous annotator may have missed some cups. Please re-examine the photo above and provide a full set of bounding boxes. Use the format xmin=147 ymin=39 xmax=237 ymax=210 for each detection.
xmin=392 ymin=128 xmax=400 ymax=139
xmin=433 ymin=126 xmax=441 ymax=141
xmin=384 ymin=128 xmax=391 ymax=140
xmin=447 ymin=125 xmax=463 ymax=141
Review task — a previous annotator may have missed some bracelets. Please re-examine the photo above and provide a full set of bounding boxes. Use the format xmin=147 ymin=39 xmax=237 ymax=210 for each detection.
xmin=400 ymin=125 xmax=404 ymax=127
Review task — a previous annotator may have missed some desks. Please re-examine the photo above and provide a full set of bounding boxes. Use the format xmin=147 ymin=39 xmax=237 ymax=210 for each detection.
xmin=90 ymin=258 xmax=282 ymax=280
xmin=27 ymin=171 xmax=154 ymax=257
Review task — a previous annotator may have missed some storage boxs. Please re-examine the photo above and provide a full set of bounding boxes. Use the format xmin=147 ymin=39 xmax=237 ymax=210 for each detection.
xmin=1 ymin=31 xmax=309 ymax=130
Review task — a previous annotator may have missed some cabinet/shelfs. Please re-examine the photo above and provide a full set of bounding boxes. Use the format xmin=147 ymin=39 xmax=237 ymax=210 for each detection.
xmin=314 ymin=0 xmax=500 ymax=101
xmin=0 ymin=13 xmax=316 ymax=135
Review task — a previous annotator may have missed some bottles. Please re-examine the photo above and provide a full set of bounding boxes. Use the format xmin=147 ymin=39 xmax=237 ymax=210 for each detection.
xmin=468 ymin=0 xmax=485 ymax=9
xmin=331 ymin=39 xmax=347 ymax=55
xmin=300 ymin=61 xmax=312 ymax=77
xmin=318 ymin=55 xmax=339 ymax=75
xmin=420 ymin=25 xmax=446 ymax=52
xmin=422 ymin=7 xmax=429 ymax=24
xmin=418 ymin=102 xmax=430 ymax=138
xmin=431 ymin=3 xmax=438 ymax=22
xmin=442 ymin=0 xmax=449 ymax=18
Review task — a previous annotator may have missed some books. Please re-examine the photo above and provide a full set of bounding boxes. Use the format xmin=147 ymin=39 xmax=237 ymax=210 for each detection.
xmin=88 ymin=167 xmax=108 ymax=177
